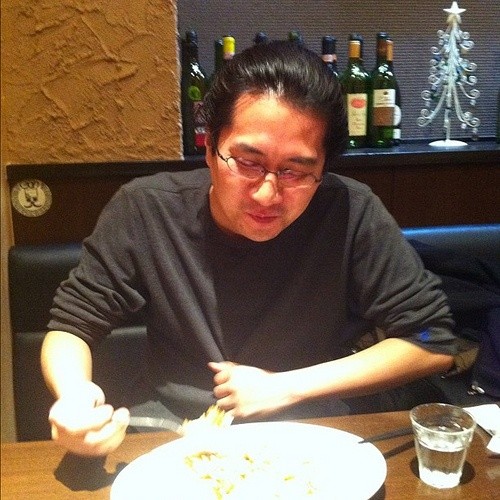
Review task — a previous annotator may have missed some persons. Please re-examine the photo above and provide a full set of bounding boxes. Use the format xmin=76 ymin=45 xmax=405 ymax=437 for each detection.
xmin=41 ymin=41 xmax=456 ymax=457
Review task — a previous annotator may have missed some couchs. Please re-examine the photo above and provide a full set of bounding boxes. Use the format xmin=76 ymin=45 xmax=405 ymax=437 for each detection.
xmin=10 ymin=223 xmax=500 ymax=441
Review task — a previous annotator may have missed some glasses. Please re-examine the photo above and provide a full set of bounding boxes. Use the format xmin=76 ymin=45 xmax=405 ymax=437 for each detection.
xmin=212 ymin=137 xmax=325 ymax=191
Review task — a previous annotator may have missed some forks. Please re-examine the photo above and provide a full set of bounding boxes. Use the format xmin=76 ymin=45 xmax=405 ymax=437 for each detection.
xmin=129 ymin=416 xmax=235 ymax=442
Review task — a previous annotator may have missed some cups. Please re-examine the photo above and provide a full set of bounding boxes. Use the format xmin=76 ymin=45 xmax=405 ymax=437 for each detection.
xmin=409 ymin=403 xmax=476 ymax=489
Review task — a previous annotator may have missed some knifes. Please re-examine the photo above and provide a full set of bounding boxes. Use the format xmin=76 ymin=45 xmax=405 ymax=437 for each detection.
xmin=358 ymin=416 xmax=460 ymax=445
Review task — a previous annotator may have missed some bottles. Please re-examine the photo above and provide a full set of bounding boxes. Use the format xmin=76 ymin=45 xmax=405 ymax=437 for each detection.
xmin=370 ymin=32 xmax=402 ymax=149
xmin=320 ymin=36 xmax=339 ymax=77
xmin=339 ymin=32 xmax=370 ymax=149
xmin=208 ymin=35 xmax=235 ymax=86
xmin=289 ymin=32 xmax=305 ymax=47
xmin=254 ymin=32 xmax=267 ymax=45
xmin=179 ymin=30 xmax=208 ymax=156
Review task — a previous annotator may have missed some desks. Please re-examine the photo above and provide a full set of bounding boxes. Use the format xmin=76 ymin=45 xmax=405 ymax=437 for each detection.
xmin=0 ymin=402 xmax=500 ymax=500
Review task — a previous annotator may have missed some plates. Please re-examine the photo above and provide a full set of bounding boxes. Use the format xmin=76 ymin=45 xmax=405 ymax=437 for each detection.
xmin=109 ymin=421 xmax=387 ymax=500
